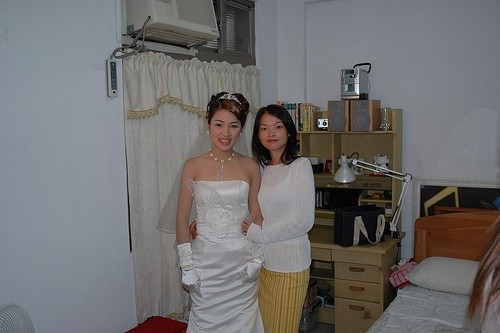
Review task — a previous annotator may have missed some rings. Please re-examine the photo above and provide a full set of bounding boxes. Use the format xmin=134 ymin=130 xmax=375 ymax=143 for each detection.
xmin=244 ymin=231 xmax=246 ymax=235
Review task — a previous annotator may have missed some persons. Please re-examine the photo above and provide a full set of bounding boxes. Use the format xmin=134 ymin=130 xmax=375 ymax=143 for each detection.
xmin=189 ymin=104 xmax=314 ymax=333
xmin=466 ymin=214 xmax=500 ymax=327
xmin=176 ymin=91 xmax=265 ymax=333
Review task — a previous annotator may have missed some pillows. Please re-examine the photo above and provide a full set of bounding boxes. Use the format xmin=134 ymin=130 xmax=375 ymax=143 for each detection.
xmin=405 ymin=256 xmax=480 ymax=295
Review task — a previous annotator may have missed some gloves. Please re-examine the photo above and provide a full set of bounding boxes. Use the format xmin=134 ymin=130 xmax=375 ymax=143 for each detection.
xmin=241 ymin=243 xmax=264 ymax=284
xmin=177 ymin=242 xmax=200 ymax=293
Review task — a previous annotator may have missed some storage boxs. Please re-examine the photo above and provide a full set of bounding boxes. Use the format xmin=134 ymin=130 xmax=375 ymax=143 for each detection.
xmin=298 ymin=296 xmax=327 ymax=333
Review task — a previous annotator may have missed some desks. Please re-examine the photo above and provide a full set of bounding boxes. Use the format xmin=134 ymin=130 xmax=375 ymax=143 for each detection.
xmin=308 ymin=228 xmax=406 ymax=333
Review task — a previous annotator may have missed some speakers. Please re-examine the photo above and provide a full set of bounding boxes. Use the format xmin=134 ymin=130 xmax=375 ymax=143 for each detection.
xmin=350 ymin=100 xmax=381 ymax=132
xmin=339 ymin=68 xmax=370 ymax=100
xmin=328 ymin=100 xmax=349 ymax=132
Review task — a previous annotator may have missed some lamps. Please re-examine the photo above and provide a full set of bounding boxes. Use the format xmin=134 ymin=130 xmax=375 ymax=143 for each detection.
xmin=333 ymin=153 xmax=412 ymax=237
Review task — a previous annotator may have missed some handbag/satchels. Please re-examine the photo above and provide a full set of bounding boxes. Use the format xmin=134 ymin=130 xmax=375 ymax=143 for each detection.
xmin=334 ymin=203 xmax=385 ymax=247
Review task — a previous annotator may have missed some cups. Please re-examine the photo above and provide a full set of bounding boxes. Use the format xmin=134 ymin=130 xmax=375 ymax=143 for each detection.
xmin=379 ymin=107 xmax=391 ymax=130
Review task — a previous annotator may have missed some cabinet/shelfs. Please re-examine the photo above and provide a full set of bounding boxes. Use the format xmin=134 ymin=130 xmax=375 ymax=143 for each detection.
xmin=296 ymin=109 xmax=403 ymax=238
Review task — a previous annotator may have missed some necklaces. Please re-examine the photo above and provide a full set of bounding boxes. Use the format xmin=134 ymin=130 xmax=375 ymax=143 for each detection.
xmin=208 ymin=147 xmax=238 ymax=181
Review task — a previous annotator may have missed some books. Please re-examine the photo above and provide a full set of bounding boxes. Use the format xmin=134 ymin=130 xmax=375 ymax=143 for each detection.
xmin=276 ymin=100 xmax=322 ymax=132
xmin=315 ymin=189 xmax=332 ymax=208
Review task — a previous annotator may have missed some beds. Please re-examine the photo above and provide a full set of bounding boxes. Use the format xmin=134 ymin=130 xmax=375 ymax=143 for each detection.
xmin=364 ymin=211 xmax=500 ymax=333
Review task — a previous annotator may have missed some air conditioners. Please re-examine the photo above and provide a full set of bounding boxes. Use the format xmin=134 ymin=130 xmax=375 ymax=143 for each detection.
xmin=125 ymin=0 xmax=221 ymax=47
